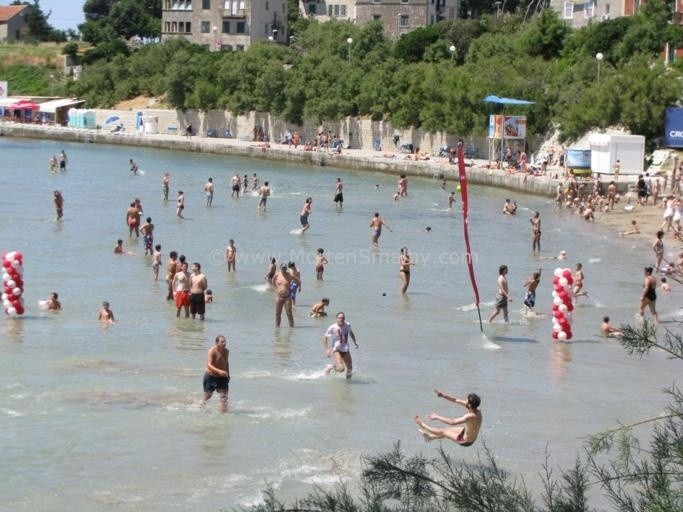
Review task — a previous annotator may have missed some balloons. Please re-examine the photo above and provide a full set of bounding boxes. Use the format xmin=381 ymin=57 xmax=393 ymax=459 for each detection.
xmin=0 ymin=251 xmax=24 ymax=318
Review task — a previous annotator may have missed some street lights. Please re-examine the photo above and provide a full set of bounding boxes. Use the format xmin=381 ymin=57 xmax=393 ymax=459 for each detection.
xmin=271 ymin=28 xmax=277 ymax=39
xmin=288 ymin=35 xmax=294 ymax=49
xmin=212 ymin=26 xmax=219 ymax=51
xmin=594 ymin=52 xmax=603 ymax=86
xmin=449 ymin=45 xmax=456 ymax=62
xmin=345 ymin=37 xmax=354 ymax=62
